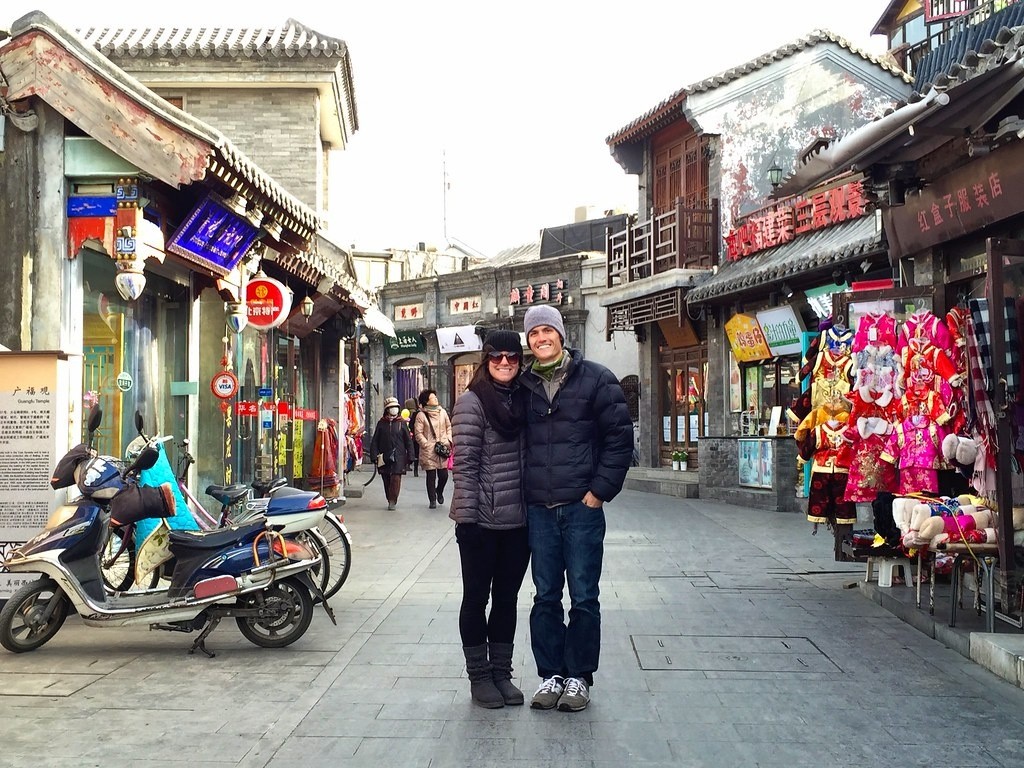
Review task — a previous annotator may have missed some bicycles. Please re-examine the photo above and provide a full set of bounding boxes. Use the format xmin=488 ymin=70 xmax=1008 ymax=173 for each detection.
xmin=343 ymin=448 xmax=378 ymax=487
xmin=96 ymin=436 xmax=354 ymax=604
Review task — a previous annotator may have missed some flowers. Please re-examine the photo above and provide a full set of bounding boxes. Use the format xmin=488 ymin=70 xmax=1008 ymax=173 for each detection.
xmin=670 ymin=449 xmax=688 ymax=462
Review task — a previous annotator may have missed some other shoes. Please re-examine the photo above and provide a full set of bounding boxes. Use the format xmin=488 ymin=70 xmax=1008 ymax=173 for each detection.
xmin=429 ymin=494 xmax=436 ymax=509
xmin=435 ymin=488 xmax=444 ymax=504
xmin=406 ymin=467 xmax=412 ymax=471
xmin=414 ymin=472 xmax=418 ymax=477
xmin=388 ymin=500 xmax=395 ymax=509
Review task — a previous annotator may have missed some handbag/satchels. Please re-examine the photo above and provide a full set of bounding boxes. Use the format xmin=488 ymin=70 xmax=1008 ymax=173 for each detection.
xmin=377 ymin=451 xmax=395 ymax=468
xmin=434 ymin=442 xmax=450 ymax=458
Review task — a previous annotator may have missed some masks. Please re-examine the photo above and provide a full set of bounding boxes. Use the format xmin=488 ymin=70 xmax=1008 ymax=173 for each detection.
xmin=387 ymin=407 xmax=399 ymax=415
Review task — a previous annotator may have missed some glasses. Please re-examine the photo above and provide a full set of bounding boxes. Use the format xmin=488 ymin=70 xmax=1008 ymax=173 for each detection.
xmin=488 ymin=352 xmax=520 ymax=364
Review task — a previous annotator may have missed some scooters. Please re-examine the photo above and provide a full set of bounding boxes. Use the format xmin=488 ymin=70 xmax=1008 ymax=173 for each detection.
xmin=0 ymin=405 xmax=339 ymax=659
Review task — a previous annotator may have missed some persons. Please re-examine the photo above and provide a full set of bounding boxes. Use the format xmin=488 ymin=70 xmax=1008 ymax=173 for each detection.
xmin=448 ymin=330 xmax=532 ymax=707
xmin=402 ymin=398 xmax=422 ymax=476
xmin=518 ymin=304 xmax=634 ymax=712
xmin=370 ymin=397 xmax=415 ymax=509
xmin=414 ymin=389 xmax=452 ymax=508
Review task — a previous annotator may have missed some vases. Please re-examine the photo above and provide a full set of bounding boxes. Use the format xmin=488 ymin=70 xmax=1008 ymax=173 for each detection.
xmin=680 ymin=461 xmax=687 ymax=472
xmin=673 ymin=461 xmax=679 ymax=470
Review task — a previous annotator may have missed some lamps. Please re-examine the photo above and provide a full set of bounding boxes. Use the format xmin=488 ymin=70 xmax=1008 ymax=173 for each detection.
xmin=261 ymin=219 xmax=283 ymax=243
xmin=832 ymin=258 xmax=872 ymax=287
xmin=345 ymin=320 xmax=356 ymax=339
xmin=993 ymin=115 xmax=1024 ymax=141
xmin=300 ymin=288 xmax=314 ymax=324
xmin=332 ymin=313 xmax=342 ymax=336
xmin=245 ymin=205 xmax=264 ymax=228
xmin=767 ymin=161 xmax=783 ymax=186
xmin=283 ymin=274 xmax=294 ymax=305
xmin=221 ymin=191 xmax=247 ymax=215
xmin=734 ymin=302 xmax=744 ymax=314
xmin=769 ymin=282 xmax=793 ymax=307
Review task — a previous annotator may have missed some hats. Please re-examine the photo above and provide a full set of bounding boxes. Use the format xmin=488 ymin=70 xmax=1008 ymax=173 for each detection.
xmin=482 ymin=330 xmax=523 ymax=360
xmin=383 ymin=398 xmax=400 ymax=409
xmin=524 ymin=305 xmax=565 ymax=352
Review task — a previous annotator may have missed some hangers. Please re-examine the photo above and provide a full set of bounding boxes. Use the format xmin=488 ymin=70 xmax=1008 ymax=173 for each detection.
xmin=826 ymin=294 xmax=967 ymax=409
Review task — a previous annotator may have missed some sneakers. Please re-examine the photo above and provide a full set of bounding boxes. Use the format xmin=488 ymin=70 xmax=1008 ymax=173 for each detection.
xmin=557 ymin=676 xmax=590 ymax=712
xmin=529 ymin=675 xmax=564 ymax=709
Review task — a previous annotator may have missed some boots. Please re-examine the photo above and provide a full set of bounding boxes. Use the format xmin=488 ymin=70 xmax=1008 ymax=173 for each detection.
xmin=462 ymin=643 xmax=505 ymax=708
xmin=488 ymin=643 xmax=524 ymax=704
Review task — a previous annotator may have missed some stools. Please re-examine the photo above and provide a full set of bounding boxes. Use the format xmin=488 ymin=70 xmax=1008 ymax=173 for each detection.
xmin=949 ymin=554 xmax=999 ymax=633
xmin=865 ymin=556 xmax=914 ymax=588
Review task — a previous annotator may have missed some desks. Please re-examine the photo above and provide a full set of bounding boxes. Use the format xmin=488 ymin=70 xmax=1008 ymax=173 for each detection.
xmin=913 ymin=544 xmax=1024 ymax=616
